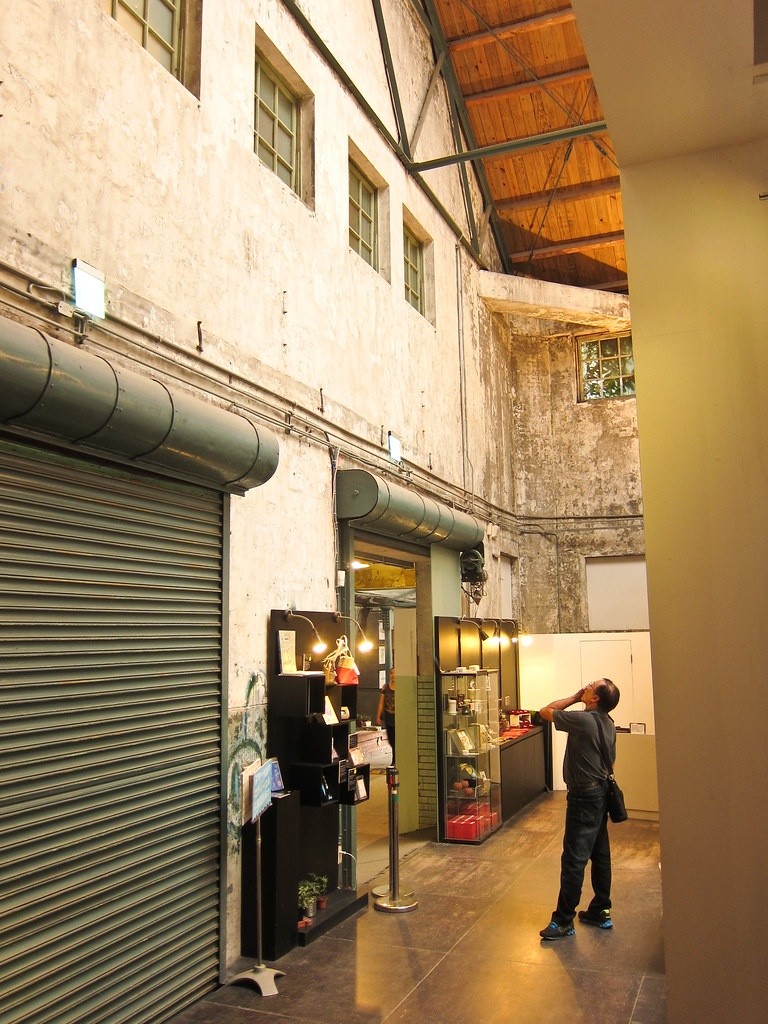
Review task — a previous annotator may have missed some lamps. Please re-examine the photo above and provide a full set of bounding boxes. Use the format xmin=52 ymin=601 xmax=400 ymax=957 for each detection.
xmin=334 ymin=611 xmax=370 ymax=644
xmin=457 ymin=618 xmax=489 ymax=641
xmin=501 ymin=620 xmax=518 ymax=641
xmin=481 ymin=620 xmax=499 ymax=639
xmin=284 ymin=610 xmax=328 ymax=653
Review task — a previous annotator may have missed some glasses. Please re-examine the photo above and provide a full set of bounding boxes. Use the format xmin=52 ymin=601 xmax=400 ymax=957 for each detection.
xmin=587 ymin=681 xmax=599 ymax=697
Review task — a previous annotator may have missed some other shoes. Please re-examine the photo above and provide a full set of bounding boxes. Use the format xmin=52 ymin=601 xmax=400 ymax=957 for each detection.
xmin=380 ymin=770 xmax=387 ymax=775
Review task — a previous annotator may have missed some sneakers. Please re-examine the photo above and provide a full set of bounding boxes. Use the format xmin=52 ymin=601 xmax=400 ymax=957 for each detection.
xmin=579 ymin=909 xmax=613 ymax=928
xmin=540 ymin=921 xmax=574 ymax=940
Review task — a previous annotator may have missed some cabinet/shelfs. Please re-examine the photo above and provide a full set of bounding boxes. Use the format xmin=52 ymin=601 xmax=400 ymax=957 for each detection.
xmin=269 ymin=609 xmax=370 ymax=947
xmin=434 ymin=616 xmax=553 ymax=821
xmin=438 ymin=669 xmax=504 ymax=846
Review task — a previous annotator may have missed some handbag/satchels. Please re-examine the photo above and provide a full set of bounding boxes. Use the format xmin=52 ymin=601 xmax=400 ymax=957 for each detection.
xmin=605 ymin=776 xmax=628 ymax=823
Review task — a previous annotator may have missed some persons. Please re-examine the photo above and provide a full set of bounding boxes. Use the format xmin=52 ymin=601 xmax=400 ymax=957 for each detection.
xmin=377 ymin=668 xmax=395 ymax=766
xmin=539 ymin=678 xmax=621 ymax=941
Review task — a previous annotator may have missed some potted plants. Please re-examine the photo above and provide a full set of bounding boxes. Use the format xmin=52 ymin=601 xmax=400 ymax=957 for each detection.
xmin=298 ymin=872 xmax=329 ymax=916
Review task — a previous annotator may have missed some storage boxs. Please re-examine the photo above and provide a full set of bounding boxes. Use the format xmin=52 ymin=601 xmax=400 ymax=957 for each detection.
xmin=510 ymin=715 xmax=531 ymax=727
xmin=447 ymin=802 xmax=499 ymax=840
